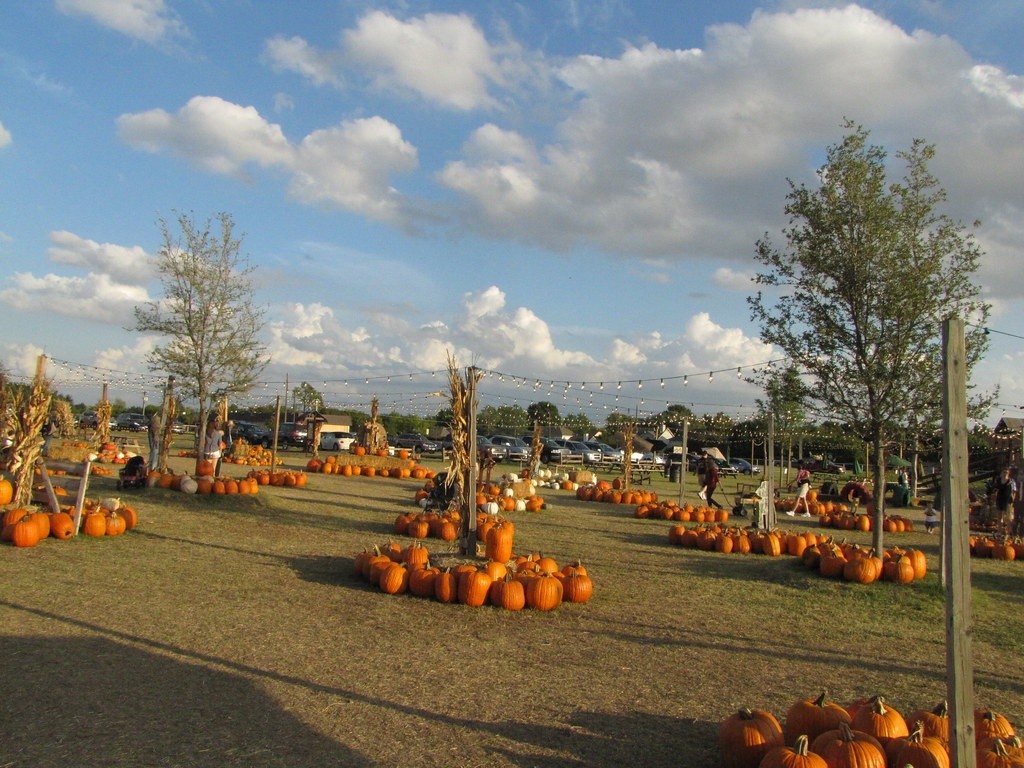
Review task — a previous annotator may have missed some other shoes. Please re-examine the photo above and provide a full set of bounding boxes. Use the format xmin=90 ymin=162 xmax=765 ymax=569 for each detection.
xmin=702 ymin=496 xmax=706 ymax=499
xmin=697 ymin=492 xmax=703 ymax=500
xmin=786 ymin=511 xmax=795 ymax=516
xmin=801 ymin=513 xmax=810 ymax=517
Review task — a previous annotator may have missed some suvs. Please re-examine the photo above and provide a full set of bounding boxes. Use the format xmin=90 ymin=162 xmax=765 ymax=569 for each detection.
xmin=117 ymin=413 xmax=146 ymax=431
xmin=278 ymin=422 xmax=308 ymax=450
xmin=221 ymin=420 xmax=273 ymax=449
xmin=394 ymin=433 xmax=437 ymax=455
xmin=78 ymin=410 xmax=118 ymax=431
xmin=464 ymin=435 xmax=507 ymax=464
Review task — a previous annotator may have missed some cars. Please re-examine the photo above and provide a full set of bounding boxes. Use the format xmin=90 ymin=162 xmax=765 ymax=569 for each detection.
xmin=726 ymin=458 xmax=761 ymax=476
xmin=441 ymin=433 xmax=453 ymax=450
xmin=424 ymin=435 xmax=443 ymax=452
xmin=639 ymin=454 xmax=665 ymax=466
xmin=773 ymin=456 xmax=799 ymax=468
xmin=615 ymin=445 xmax=645 ymax=463
xmin=488 ymin=435 xmax=533 ymax=463
xmin=797 ymin=457 xmax=845 ymax=475
xmin=666 ymin=453 xmax=703 ymax=473
xmin=317 ymin=431 xmax=356 ymax=452
xmin=161 ymin=421 xmax=186 ymax=434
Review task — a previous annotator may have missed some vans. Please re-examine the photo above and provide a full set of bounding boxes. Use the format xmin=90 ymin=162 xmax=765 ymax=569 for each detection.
xmin=554 ymin=440 xmax=602 ymax=465
xmin=583 ymin=442 xmax=625 ymax=467
xmin=521 ymin=436 xmax=572 ymax=464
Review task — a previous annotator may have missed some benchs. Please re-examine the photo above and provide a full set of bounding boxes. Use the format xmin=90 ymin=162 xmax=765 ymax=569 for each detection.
xmin=113 ymin=443 xmax=143 ymax=454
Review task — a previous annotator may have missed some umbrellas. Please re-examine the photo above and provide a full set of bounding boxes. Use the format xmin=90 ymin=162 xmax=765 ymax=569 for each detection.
xmin=701 ymin=446 xmax=726 ymax=461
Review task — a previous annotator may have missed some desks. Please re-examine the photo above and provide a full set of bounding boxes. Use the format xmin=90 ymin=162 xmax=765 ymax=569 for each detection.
xmin=111 ymin=435 xmax=142 ymax=454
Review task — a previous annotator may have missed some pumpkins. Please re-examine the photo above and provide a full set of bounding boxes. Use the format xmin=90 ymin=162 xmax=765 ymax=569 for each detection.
xmin=91 ymin=464 xmax=112 ymax=475
xmin=0 ymin=475 xmax=137 ymax=547
xmin=178 ymin=437 xmax=282 ymax=467
xmin=306 ymin=440 xmax=436 ymax=480
xmin=146 ymin=460 xmax=307 ymax=495
xmin=774 ymin=491 xmax=913 ymax=533
xmin=355 ymin=488 xmax=593 ymax=611
xmin=62 ymin=442 xmax=87 ymax=447
xmin=475 ymin=468 xmax=598 ymax=515
xmin=34 ymin=468 xmax=64 ymax=476
xmin=575 ymin=481 xmax=728 ymax=522
xmin=87 ymin=442 xmax=137 ymax=464
xmin=968 ymin=532 xmax=1024 ymax=561
xmin=716 ymin=690 xmax=1024 ymax=768
xmin=668 ymin=522 xmax=926 ymax=585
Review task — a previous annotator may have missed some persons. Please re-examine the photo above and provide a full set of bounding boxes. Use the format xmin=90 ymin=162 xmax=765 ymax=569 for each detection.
xmin=785 ymin=459 xmax=811 ymax=517
xmin=41 ymin=421 xmax=56 ymax=456
xmin=696 ymin=451 xmax=707 ymax=500
xmin=924 ymin=503 xmax=937 ymax=533
xmin=990 ymin=466 xmax=1024 ymax=540
xmin=204 ymin=413 xmax=233 ymax=476
xmin=146 ymin=409 xmax=161 ymax=471
xmin=4 ymin=430 xmax=16 ymax=446
xmin=703 ymin=455 xmax=722 ymax=509
